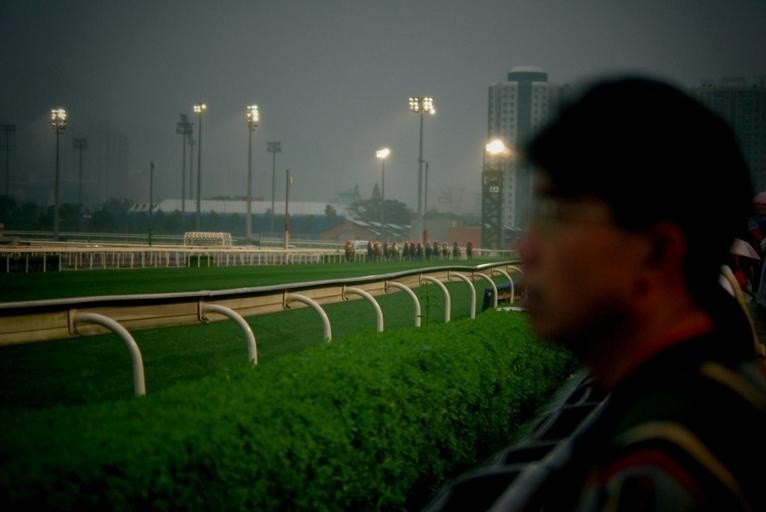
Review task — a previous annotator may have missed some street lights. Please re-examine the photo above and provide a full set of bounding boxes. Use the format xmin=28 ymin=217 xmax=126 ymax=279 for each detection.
xmin=176 ymin=102 xmax=207 ymax=230
xmin=408 ymin=95 xmax=436 ymax=243
xmin=375 ymin=148 xmax=391 ymax=241
xmin=245 ymin=104 xmax=263 ymax=240
xmin=48 ymin=106 xmax=68 ymax=240
xmin=266 ymin=140 xmax=281 ymax=238
xmin=73 ymin=135 xmax=88 ymax=201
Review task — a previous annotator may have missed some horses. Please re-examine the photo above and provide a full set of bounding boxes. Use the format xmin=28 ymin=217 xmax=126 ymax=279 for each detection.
xmin=366 ymin=241 xmax=441 ymax=263
xmin=344 ymin=244 xmax=355 ymax=264
xmin=442 ymin=243 xmax=451 ymax=263
xmin=466 ymin=245 xmax=473 ymax=263
xmin=452 ymin=246 xmax=461 ymax=263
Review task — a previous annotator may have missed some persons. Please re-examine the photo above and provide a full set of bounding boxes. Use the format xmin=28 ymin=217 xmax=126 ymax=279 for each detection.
xmin=343 ymin=239 xmax=475 ymax=265
xmin=515 ymin=74 xmax=766 ymax=510
